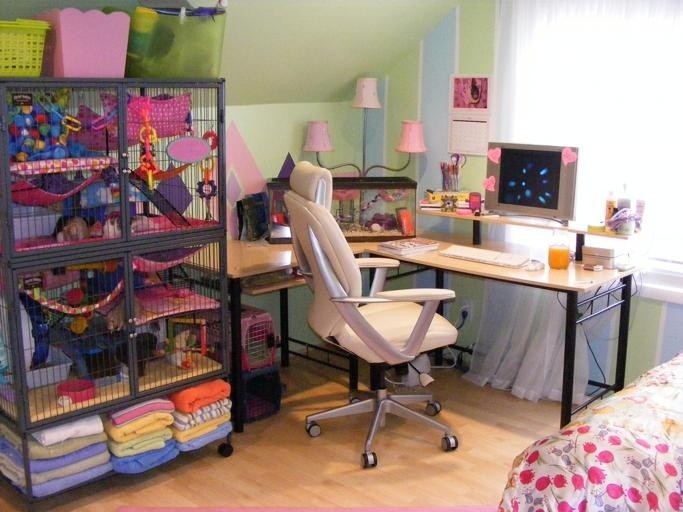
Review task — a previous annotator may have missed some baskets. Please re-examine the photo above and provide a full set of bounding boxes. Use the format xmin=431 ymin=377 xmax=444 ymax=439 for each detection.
xmin=0 ymin=18 xmax=51 ymax=77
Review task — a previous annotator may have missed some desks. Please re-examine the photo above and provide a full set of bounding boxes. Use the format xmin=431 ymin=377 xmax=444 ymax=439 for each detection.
xmin=362 ymin=232 xmax=641 ymax=432
xmin=172 ymin=228 xmax=449 ymax=434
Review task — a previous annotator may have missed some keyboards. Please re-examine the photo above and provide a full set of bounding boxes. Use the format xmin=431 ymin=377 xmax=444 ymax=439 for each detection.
xmin=440 ymin=244 xmax=530 ymax=268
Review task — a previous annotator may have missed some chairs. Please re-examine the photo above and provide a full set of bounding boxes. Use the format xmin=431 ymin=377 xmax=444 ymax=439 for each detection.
xmin=282 ymin=161 xmax=460 ymax=468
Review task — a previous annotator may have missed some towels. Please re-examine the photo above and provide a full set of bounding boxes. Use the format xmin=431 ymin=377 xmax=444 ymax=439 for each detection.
xmin=0 ymin=378 xmax=233 ymax=501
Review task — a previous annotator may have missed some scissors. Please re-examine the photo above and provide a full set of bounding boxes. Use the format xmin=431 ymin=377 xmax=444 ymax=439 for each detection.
xmin=451 ymin=153 xmax=467 ymax=169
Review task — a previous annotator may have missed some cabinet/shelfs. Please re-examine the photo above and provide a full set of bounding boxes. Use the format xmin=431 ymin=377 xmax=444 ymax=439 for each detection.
xmin=0 ymin=76 xmax=234 ymax=504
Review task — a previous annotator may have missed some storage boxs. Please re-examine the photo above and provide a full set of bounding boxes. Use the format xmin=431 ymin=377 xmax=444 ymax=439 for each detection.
xmin=32 ymin=5 xmax=228 ymax=78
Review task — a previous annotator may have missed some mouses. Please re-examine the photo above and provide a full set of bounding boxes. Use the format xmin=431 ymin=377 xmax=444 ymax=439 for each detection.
xmin=527 ymin=260 xmax=544 ymax=270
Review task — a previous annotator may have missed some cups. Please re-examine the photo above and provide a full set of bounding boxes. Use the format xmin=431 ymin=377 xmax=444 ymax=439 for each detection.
xmin=549 ymin=242 xmax=569 ymax=269
xmin=442 ymin=172 xmax=459 ymax=191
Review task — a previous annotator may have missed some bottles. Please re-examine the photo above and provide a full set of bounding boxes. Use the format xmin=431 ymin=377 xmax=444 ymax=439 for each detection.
xmin=605 ymin=181 xmax=631 ymax=229
xmin=128 ymin=6 xmax=159 ymax=62
xmin=469 ymin=192 xmax=481 ymax=213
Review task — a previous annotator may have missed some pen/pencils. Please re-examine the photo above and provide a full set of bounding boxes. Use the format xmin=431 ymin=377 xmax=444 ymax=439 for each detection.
xmin=440 ymin=162 xmax=459 ymax=191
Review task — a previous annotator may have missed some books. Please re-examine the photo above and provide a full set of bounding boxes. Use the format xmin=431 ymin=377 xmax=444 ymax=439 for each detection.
xmin=382 ymin=237 xmax=438 ymax=250
xmin=378 ymin=243 xmax=438 ymax=255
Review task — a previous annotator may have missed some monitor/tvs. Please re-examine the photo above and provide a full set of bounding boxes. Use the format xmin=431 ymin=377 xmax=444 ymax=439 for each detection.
xmin=484 ymin=140 xmax=579 ymax=224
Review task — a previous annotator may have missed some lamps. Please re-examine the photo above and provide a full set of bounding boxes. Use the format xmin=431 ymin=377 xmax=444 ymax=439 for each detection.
xmin=301 ymin=79 xmax=429 ymax=229
